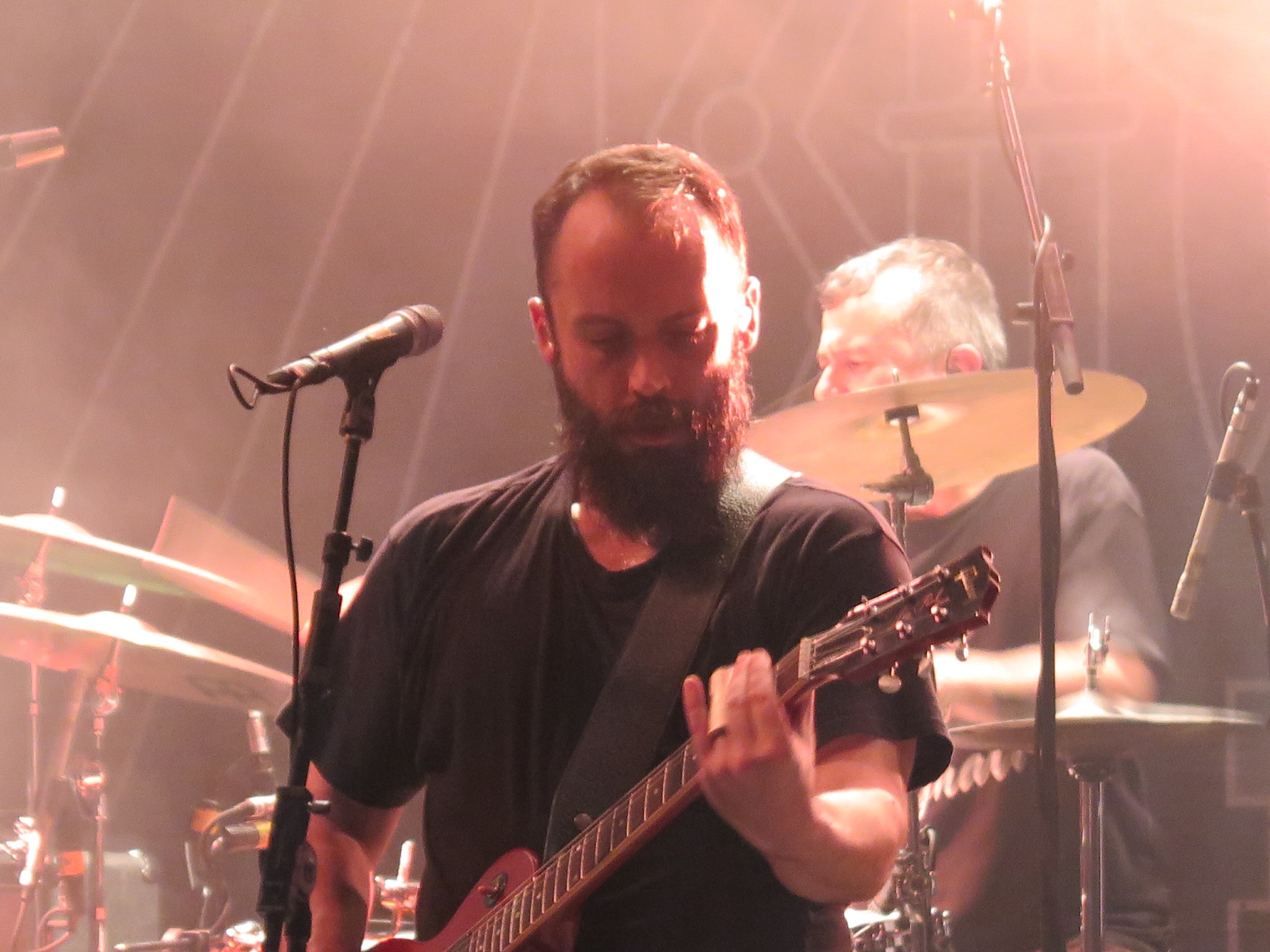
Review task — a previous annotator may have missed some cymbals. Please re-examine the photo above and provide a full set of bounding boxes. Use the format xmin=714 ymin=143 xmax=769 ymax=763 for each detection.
xmin=1 ymin=600 xmax=296 ymax=715
xmin=944 ymin=690 xmax=1260 ymax=764
xmin=0 ymin=509 xmax=258 ymax=596
xmin=739 ymin=364 xmax=1149 ymax=502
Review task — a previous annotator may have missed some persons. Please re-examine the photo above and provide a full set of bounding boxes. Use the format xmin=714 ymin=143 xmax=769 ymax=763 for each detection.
xmin=812 ymin=236 xmax=1170 ymax=951
xmin=273 ymin=142 xmax=954 ymax=952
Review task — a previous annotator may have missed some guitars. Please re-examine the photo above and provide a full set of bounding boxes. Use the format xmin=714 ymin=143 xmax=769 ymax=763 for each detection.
xmin=366 ymin=538 xmax=1005 ymax=952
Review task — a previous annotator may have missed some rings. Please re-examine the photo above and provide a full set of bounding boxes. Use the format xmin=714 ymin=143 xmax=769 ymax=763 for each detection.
xmin=705 ymin=721 xmax=722 ymax=751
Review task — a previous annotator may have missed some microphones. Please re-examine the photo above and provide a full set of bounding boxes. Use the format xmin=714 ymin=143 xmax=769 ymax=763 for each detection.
xmin=252 ymin=301 xmax=446 ymax=396
xmin=1167 ymin=376 xmax=1261 ymax=623
